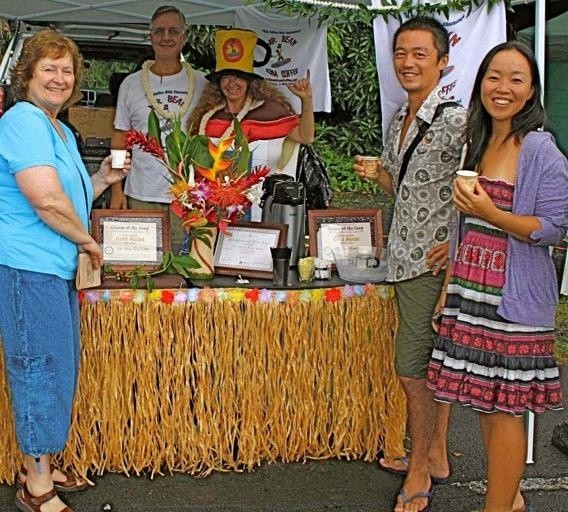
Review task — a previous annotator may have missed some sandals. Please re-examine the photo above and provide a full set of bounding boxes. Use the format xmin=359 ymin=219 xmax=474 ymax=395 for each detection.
xmin=14 ymin=480 xmax=75 ymax=512
xmin=16 ymin=461 xmax=89 ymax=494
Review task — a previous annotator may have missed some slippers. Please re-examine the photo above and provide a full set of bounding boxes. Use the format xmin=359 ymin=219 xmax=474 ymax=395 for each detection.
xmin=378 ymin=455 xmax=453 ymax=484
xmin=393 ymin=481 xmax=440 ymax=510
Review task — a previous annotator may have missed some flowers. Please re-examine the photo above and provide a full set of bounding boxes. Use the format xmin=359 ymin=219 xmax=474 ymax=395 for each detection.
xmin=123 ymin=108 xmax=271 ymax=239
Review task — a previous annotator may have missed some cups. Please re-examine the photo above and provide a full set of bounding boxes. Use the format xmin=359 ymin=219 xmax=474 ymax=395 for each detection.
xmin=455 ymin=170 xmax=480 ymax=195
xmin=272 ymin=259 xmax=291 ymax=288
xmin=110 ymin=149 xmax=127 ymax=170
xmin=361 ymin=156 xmax=378 ymax=180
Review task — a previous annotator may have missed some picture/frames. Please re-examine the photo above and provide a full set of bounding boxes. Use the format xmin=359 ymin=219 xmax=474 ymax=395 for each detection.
xmin=212 ymin=220 xmax=289 ymax=280
xmin=92 ymin=209 xmax=170 ymax=274
xmin=308 ymin=209 xmax=383 ymax=270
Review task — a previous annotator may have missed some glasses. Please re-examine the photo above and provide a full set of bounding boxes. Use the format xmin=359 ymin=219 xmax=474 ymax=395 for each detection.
xmin=151 ymin=27 xmax=183 ymax=38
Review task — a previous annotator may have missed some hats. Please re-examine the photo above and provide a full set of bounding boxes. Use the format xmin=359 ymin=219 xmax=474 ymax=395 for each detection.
xmin=204 ymin=28 xmax=265 ymax=84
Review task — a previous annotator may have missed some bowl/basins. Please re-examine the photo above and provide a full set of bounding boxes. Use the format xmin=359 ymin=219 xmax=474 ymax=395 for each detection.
xmin=325 ymin=244 xmax=389 ymax=283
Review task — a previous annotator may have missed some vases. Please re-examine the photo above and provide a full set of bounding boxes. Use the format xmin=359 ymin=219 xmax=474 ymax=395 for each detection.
xmin=183 ymin=223 xmax=218 ymax=280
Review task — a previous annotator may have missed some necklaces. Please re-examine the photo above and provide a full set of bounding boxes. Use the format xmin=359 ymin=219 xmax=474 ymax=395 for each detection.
xmin=141 ymin=58 xmax=197 ymax=119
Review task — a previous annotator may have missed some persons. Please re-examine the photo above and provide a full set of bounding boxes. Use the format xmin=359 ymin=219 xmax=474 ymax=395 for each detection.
xmin=352 ymin=15 xmax=471 ymax=512
xmin=196 ymin=59 xmax=315 ymax=222
xmin=0 ymin=28 xmax=132 ymax=512
xmin=426 ymin=40 xmax=568 ymax=512
xmin=109 ymin=4 xmax=211 ymax=244
